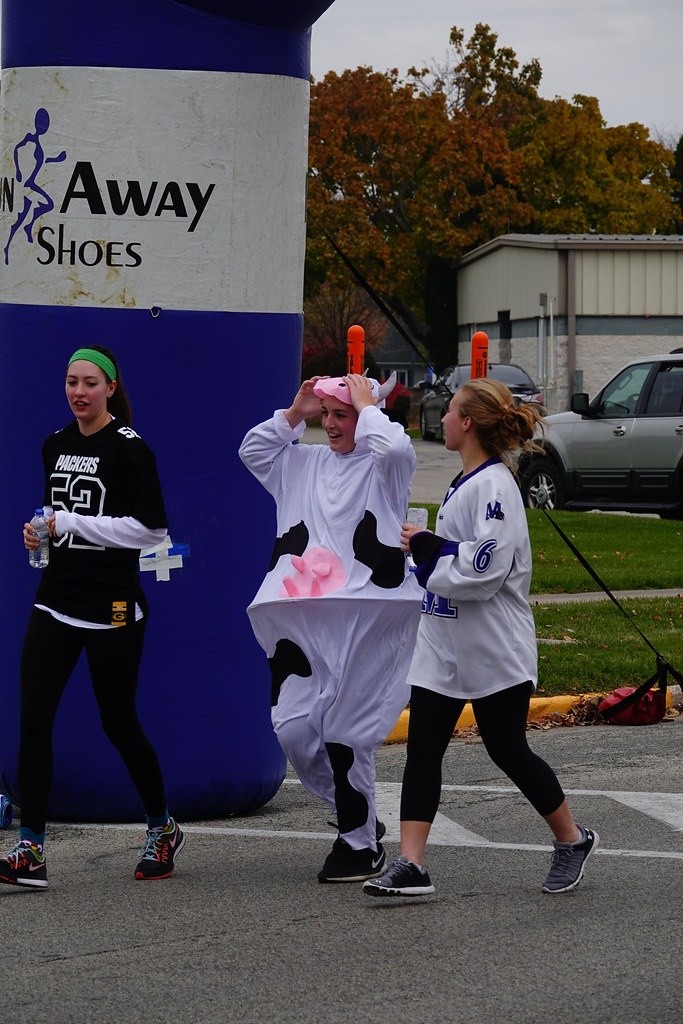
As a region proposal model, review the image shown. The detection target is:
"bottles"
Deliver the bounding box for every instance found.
[28,509,50,568]
[404,507,428,573]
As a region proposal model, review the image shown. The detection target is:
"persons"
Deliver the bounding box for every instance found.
[362,378,599,898]
[237,368,425,885]
[0,343,186,889]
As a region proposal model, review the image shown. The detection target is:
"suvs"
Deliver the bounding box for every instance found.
[515,346,683,521]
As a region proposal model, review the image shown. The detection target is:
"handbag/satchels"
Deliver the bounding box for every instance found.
[598,652,668,726]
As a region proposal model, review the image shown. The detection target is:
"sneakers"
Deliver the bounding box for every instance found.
[134,816,185,880]
[375,819,385,841]
[362,856,435,897]
[542,824,599,892]
[0,839,49,887]
[317,835,388,883]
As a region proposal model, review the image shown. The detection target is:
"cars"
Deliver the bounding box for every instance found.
[418,363,543,444]
